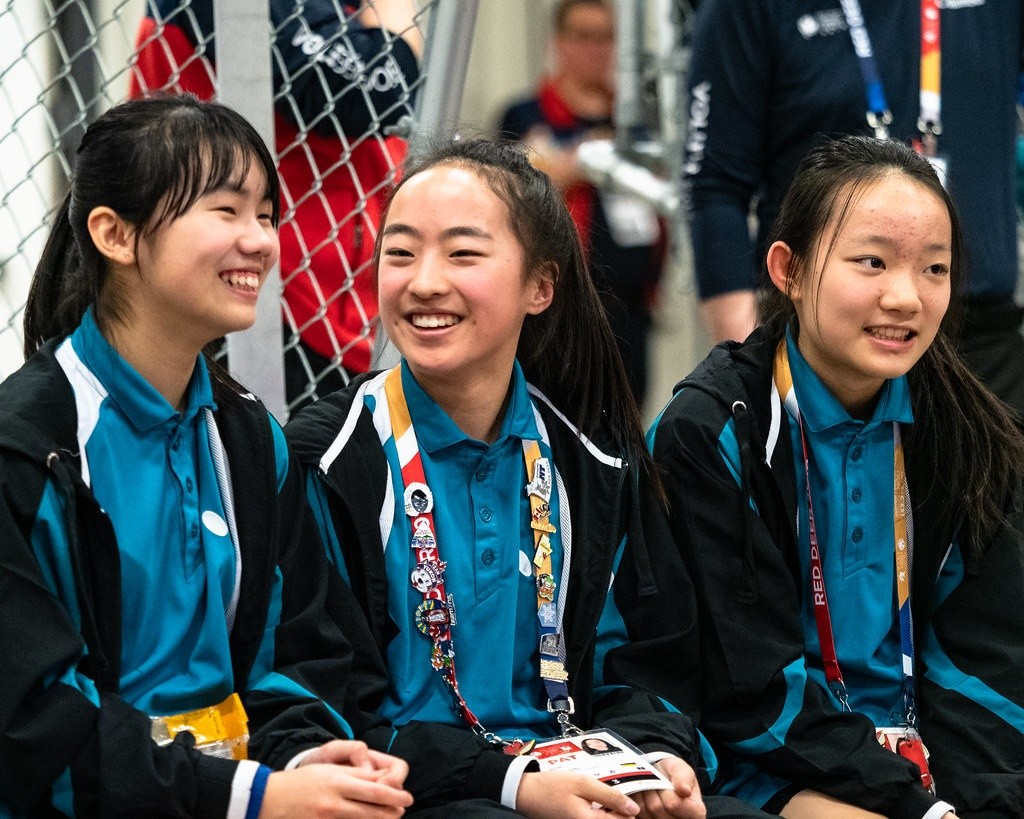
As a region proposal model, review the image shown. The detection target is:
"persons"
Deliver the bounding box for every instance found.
[0,93,412,818]
[581,738,622,755]
[624,137,1024,815]
[274,137,703,818]
[679,2,1021,464]
[487,0,677,459]
[133,0,427,444]
